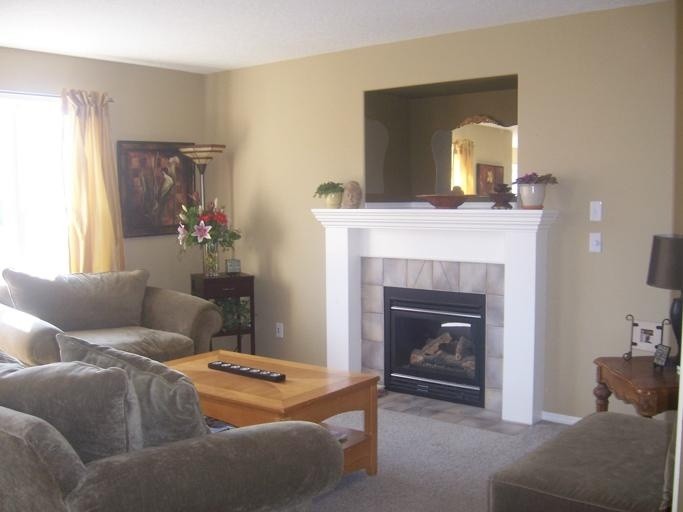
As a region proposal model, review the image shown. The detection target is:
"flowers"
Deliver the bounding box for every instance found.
[178,193,241,271]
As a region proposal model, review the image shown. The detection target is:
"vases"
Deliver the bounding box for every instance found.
[202,242,218,277]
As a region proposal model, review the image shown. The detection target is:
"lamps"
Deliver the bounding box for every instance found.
[647,235,682,365]
[178,143,226,209]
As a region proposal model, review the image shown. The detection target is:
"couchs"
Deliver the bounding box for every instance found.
[0,269,223,367]
[487,333,683,512]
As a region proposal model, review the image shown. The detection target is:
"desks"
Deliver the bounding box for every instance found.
[191,272,255,354]
[593,356,679,418]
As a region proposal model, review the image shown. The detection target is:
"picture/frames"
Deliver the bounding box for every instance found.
[116,141,194,238]
[477,164,503,195]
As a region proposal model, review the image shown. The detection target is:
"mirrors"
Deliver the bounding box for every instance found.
[430,115,518,202]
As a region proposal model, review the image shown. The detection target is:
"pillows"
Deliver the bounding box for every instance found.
[0,269,150,330]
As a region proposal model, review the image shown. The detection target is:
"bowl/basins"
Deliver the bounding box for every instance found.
[416,194,479,209]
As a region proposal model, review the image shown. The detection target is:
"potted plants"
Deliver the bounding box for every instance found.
[220,230,241,274]
[512,172,559,209]
[313,181,344,209]
